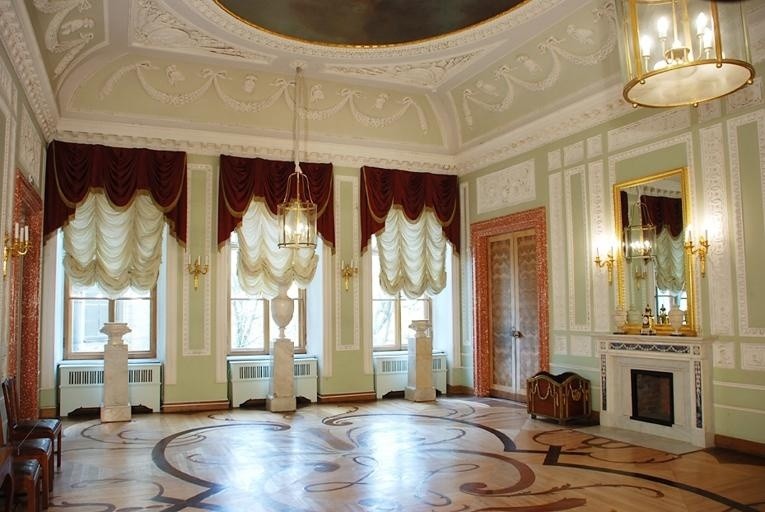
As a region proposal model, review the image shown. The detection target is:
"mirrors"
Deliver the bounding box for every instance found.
[612,167,699,335]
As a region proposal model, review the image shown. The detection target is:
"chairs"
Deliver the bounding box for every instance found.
[0,377,63,512]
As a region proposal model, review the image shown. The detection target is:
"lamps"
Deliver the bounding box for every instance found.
[2,222,30,276]
[686,227,710,278]
[275,66,317,248]
[340,258,358,292]
[615,1,756,110]
[186,254,209,291]
